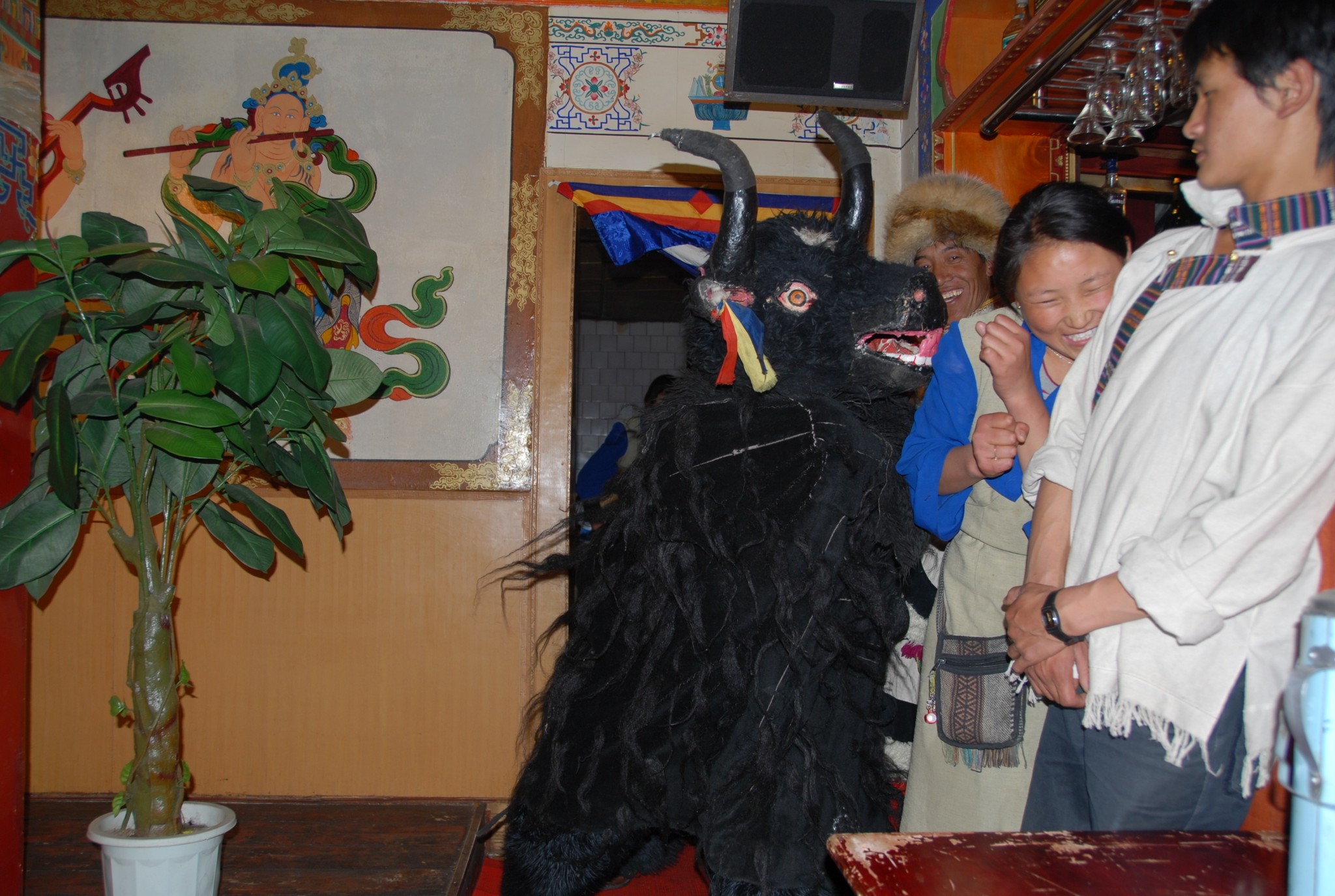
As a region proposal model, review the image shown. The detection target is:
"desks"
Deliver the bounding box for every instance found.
[825,828,1291,896]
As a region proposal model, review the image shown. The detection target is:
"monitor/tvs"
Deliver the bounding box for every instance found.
[723,0,925,112]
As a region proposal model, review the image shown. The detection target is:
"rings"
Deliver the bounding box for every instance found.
[993,444,999,461]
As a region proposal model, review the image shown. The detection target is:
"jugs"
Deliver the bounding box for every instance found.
[1286,589,1335,896]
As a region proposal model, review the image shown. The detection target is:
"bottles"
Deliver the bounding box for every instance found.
[1001,0,1032,51]
[1100,157,1127,217]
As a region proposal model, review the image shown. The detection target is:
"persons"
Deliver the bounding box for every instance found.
[882,0,1335,829]
[574,375,675,599]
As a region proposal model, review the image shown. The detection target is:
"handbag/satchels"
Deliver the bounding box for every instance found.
[924,632,1030,773]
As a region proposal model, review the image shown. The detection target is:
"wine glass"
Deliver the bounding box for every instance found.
[1065,8,1196,148]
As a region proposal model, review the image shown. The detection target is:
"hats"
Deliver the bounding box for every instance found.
[882,171,1010,267]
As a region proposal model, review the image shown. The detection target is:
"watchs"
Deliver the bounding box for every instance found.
[1040,585,1089,647]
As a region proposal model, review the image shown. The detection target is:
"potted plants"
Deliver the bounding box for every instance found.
[0,176,386,896]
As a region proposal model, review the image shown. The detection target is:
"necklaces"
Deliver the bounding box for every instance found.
[1045,345,1076,365]
